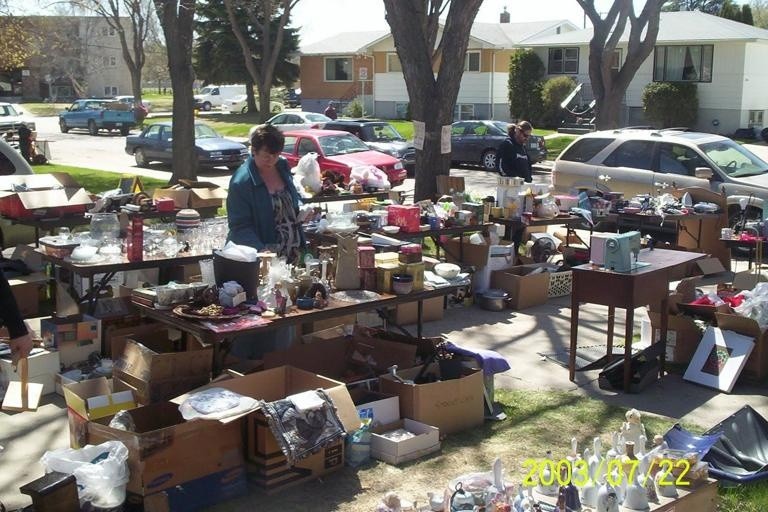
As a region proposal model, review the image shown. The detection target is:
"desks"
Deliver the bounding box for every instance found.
[531,478,718,512]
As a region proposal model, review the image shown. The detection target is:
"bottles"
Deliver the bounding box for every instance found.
[353,184,364,194]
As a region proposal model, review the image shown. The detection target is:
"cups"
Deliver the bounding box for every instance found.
[609,191,624,213]
[59,212,229,264]
[429,217,440,230]
[155,197,175,212]
[721,228,732,239]
[391,273,412,294]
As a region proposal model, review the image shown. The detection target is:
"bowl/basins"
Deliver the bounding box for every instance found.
[433,263,461,279]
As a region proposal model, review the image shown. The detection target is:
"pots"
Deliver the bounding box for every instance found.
[474,289,512,311]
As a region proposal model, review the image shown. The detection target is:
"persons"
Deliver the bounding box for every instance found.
[2,131,14,144]
[325,101,338,120]
[0,268,33,363]
[495,121,545,183]
[221,126,307,362]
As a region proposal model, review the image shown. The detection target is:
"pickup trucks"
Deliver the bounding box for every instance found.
[58,99,137,137]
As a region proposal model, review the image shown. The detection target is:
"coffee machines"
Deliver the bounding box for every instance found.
[496,176,560,221]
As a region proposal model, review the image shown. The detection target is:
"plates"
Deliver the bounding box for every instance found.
[174,302,247,320]
[326,209,401,234]
[62,357,114,382]
[623,194,651,214]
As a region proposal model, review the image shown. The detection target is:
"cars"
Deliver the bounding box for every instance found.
[248,110,332,145]
[222,95,286,115]
[450,119,546,173]
[284,87,301,108]
[125,121,249,173]
[279,129,408,193]
[0,102,38,143]
[112,95,151,111]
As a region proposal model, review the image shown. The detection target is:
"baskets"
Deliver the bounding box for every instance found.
[548,270,573,298]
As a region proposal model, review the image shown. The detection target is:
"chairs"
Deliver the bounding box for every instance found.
[681,148,703,175]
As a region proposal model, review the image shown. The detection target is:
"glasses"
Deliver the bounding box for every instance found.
[523,134,529,138]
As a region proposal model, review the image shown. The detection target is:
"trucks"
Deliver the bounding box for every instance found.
[193,83,247,112]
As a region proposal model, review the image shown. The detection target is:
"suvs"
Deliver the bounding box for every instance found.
[550,126,767,260]
[320,118,416,179]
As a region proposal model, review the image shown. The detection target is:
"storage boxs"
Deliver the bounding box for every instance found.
[647,271,767,395]
